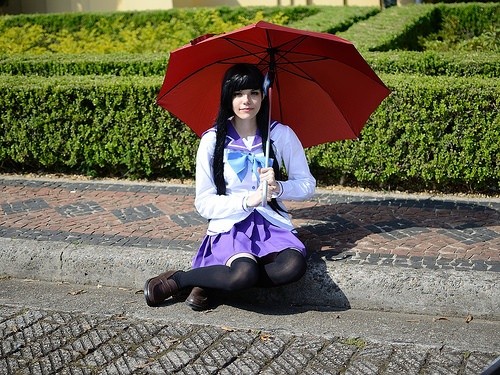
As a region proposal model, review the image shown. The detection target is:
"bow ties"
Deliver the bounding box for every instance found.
[228,151,274,184]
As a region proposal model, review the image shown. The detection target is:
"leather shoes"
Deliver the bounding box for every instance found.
[184,286,210,310]
[143,270,184,306]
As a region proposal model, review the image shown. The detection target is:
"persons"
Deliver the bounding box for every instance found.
[143,61,319,311]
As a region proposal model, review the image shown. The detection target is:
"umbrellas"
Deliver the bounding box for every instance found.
[153,19,395,209]
